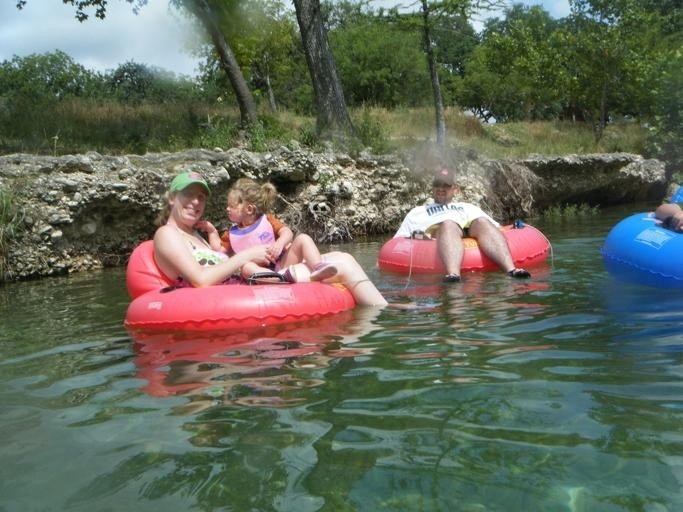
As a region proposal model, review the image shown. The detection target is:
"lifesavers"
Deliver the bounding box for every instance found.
[602,212,682,288]
[378,224,550,273]
[123,240,356,331]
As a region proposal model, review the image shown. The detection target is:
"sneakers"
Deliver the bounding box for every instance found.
[281,264,297,283]
[310,262,338,281]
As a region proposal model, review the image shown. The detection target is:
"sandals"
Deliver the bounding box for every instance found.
[442,272,462,282]
[507,267,529,277]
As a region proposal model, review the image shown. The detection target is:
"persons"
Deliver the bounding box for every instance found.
[152,170,418,309]
[190,176,337,284]
[430,286,514,385]
[374,165,533,282]
[149,332,345,446]
[654,182,683,234]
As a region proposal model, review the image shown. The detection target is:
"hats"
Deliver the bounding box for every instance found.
[169,171,211,199]
[432,168,455,186]
